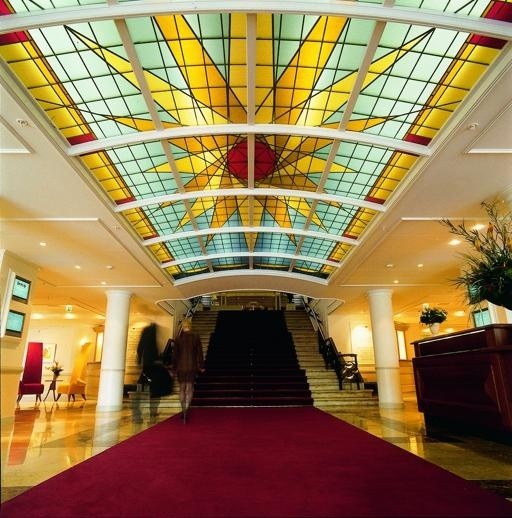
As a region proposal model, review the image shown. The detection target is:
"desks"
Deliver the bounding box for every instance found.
[43,380,63,401]
[43,401,55,418]
[411,324,512,449]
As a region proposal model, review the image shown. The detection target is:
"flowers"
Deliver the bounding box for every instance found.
[437,200,512,324]
[45,361,64,375]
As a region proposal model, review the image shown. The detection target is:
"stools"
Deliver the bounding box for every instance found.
[54,384,88,402]
[16,380,44,404]
[63,402,86,413]
[15,403,40,419]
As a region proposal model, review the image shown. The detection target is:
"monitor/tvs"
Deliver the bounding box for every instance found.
[470,308,491,327]
[12,276,32,304]
[7,308,26,338]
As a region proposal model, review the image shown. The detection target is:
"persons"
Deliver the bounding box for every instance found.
[136,322,158,416]
[173,319,206,424]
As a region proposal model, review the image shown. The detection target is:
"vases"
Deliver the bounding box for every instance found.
[53,373,58,380]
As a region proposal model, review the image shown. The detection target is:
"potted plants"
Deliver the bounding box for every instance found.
[419,305,448,336]
